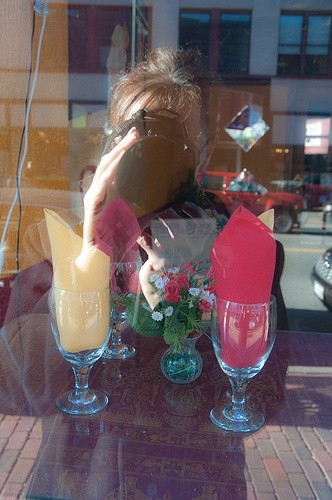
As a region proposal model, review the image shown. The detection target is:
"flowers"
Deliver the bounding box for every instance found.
[148,266,216,338]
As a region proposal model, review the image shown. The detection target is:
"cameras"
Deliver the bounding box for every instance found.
[115,119,199,210]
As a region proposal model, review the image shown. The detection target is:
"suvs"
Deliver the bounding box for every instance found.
[309,246,332,315]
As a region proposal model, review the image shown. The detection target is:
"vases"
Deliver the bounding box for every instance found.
[160,331,204,385]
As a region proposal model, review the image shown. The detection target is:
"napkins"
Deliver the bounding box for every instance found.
[43,208,110,353]
[210,205,276,369]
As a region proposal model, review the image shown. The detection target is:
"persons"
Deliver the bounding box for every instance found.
[0,47,285,500]
[294,173,302,181]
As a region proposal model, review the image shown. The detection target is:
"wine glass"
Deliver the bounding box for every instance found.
[102,255,142,359]
[210,294,277,434]
[49,286,113,415]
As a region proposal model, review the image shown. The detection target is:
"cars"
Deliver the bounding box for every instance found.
[196,170,306,234]
